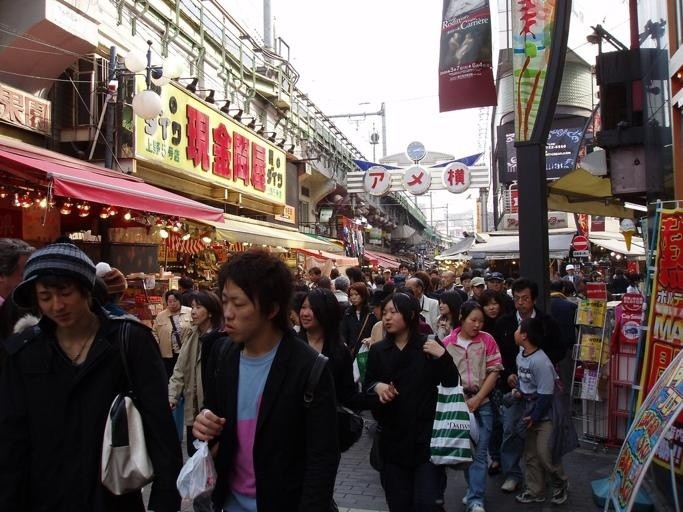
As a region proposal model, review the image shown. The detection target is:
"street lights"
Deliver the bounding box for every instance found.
[95,37,164,270]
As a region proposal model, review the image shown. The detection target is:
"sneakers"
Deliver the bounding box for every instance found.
[461,461,569,512]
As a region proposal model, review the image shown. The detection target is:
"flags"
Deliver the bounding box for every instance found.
[439,1,497,113]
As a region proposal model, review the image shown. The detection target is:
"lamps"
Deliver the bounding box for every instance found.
[109,40,183,121]
[0,184,183,231]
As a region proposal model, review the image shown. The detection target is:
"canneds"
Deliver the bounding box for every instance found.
[159,266,164,278]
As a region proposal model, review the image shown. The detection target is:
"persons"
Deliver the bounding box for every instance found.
[0,233,647,512]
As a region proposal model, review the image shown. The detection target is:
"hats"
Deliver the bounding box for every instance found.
[565,264,574,271]
[11,242,97,313]
[96,260,127,293]
[487,272,503,281]
[383,268,391,274]
[469,277,485,286]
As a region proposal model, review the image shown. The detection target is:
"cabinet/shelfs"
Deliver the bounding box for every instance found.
[568,281,644,452]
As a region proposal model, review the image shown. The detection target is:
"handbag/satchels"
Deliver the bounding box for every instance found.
[369,436,385,470]
[356,343,369,384]
[428,373,474,470]
[337,410,363,451]
[98,320,158,500]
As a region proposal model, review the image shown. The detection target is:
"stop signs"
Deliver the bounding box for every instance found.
[571,235,587,251]
